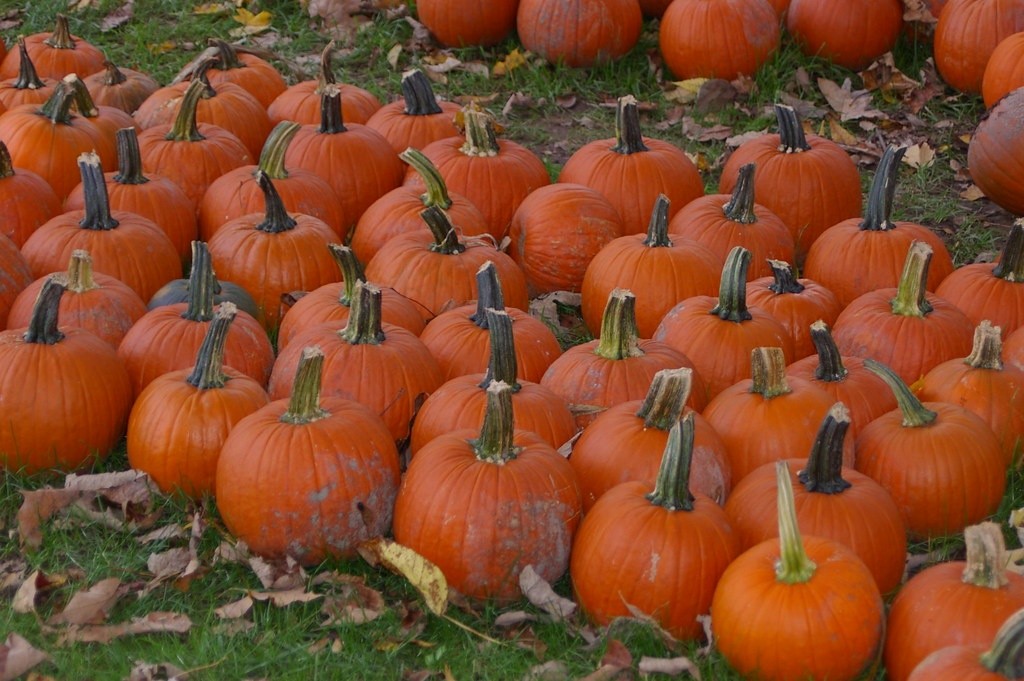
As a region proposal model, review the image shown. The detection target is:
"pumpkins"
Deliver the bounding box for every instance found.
[0,0,1024,681]
